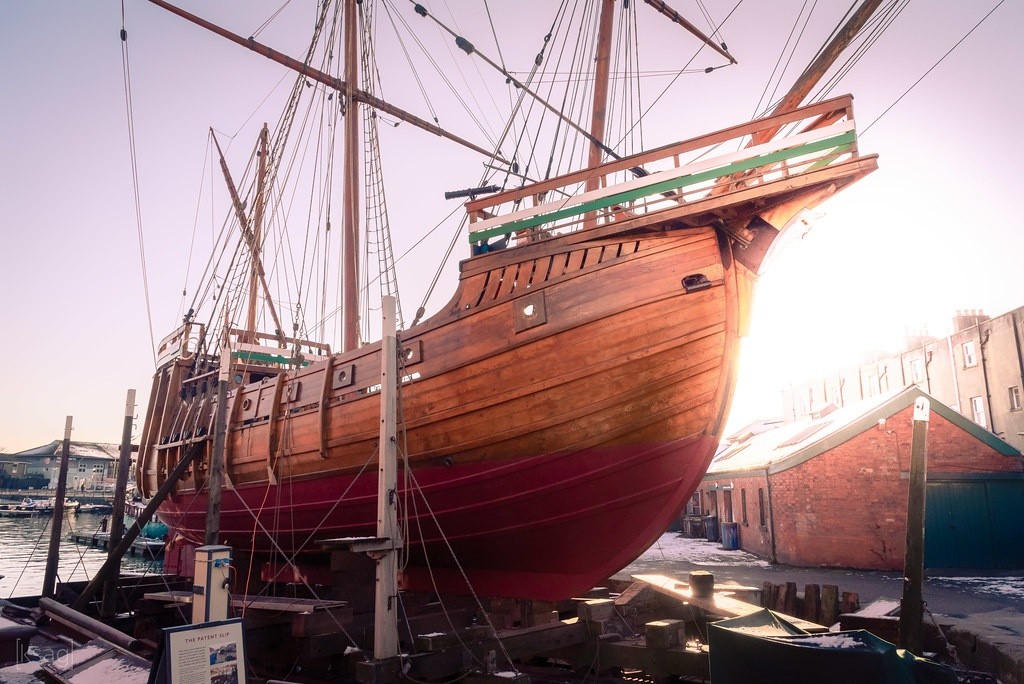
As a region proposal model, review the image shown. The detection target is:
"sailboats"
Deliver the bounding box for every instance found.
[0,1,1021,683]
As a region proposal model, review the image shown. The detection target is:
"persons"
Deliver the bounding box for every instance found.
[209,644,231,665]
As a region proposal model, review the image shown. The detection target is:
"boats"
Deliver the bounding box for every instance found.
[78,502,112,514]
[1,497,79,515]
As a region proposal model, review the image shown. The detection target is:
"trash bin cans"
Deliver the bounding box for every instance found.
[721,523,738,550]
[704,517,719,542]
